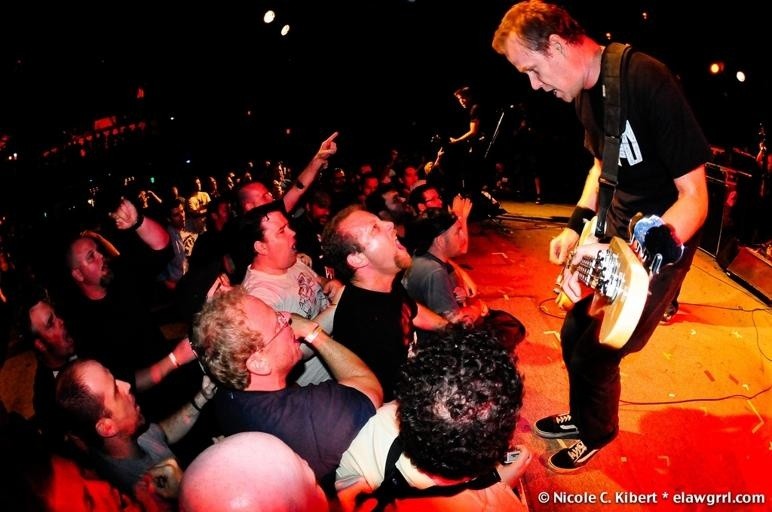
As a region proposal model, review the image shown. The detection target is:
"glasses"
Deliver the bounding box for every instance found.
[263,311,288,347]
[426,196,440,203]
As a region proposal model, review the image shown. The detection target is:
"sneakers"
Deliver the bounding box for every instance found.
[548,441,599,472]
[534,414,583,439]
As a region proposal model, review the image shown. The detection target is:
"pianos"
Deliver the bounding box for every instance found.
[705,144,762,176]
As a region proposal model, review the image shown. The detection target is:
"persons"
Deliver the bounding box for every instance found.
[494,0,711,473]
[0,129,529,512]
[446,87,490,223]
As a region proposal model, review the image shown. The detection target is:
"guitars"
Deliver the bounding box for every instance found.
[553,213,664,350]
[431,133,493,165]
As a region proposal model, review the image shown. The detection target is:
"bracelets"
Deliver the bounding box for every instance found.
[565,203,596,235]
